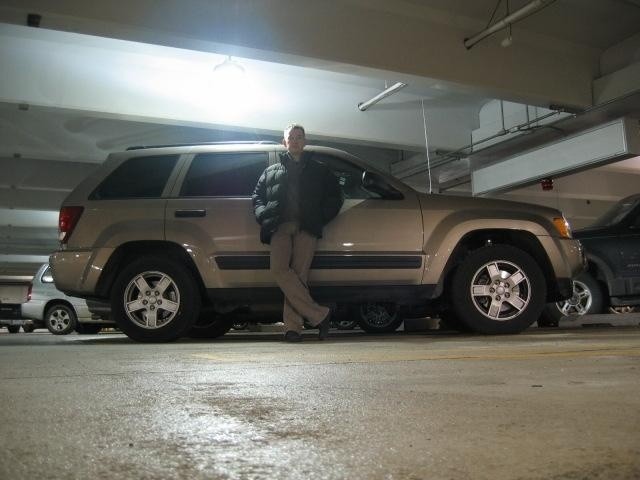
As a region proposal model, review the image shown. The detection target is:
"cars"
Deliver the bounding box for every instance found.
[231,321,360,331]
[609,306,636,314]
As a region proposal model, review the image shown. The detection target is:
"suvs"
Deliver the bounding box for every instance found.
[48,140,589,343]
[547,193,640,317]
[21,264,121,335]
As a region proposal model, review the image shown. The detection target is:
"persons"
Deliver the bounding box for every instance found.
[249,123,345,343]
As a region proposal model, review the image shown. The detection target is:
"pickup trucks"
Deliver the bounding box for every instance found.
[0,299,35,333]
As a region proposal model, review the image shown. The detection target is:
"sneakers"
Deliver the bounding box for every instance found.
[319,306,334,340]
[285,329,302,342]
